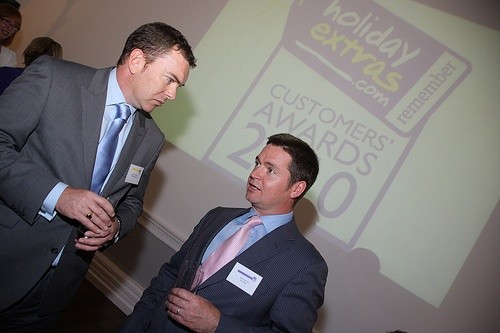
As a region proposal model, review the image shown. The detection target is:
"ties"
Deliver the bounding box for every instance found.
[189,215,263,291]
[90,103,131,194]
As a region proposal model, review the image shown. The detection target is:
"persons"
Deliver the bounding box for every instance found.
[0,21,196,333]
[115,133,328,333]
[0,0,23,68]
[0,37,63,95]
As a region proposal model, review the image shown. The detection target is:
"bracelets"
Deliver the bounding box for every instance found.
[114,218,120,239]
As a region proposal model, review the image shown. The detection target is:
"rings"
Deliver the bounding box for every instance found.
[177,308,181,314]
[87,212,94,220]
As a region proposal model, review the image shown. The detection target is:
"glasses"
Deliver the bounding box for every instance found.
[0,16,20,31]
